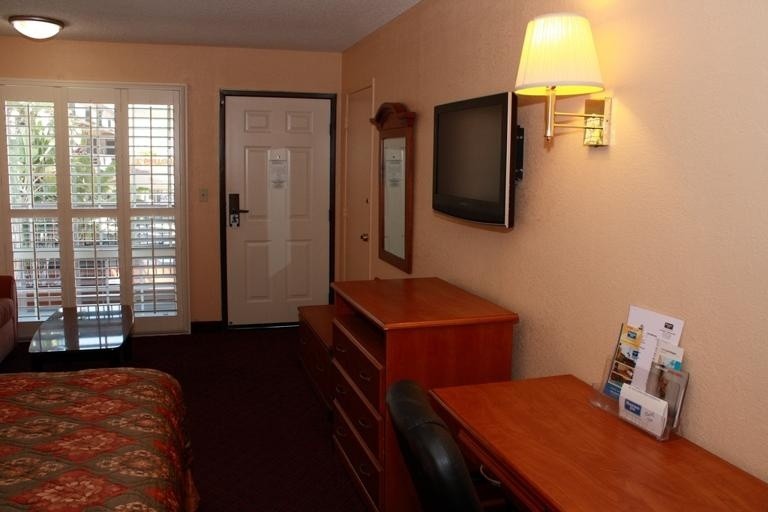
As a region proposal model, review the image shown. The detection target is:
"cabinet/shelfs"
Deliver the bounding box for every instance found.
[297,304,338,457]
[327,278,517,510]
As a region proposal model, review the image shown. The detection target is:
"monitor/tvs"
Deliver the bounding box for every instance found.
[432,90,524,229]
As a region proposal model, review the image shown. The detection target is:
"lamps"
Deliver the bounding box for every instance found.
[514,13,613,148]
[8,14,64,41]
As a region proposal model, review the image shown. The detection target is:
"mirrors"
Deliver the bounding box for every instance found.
[368,102,416,274]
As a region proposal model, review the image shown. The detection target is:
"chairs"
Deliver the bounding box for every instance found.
[1,275,19,362]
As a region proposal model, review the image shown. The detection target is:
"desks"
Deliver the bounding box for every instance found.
[27,303,135,371]
[426,373,767,512]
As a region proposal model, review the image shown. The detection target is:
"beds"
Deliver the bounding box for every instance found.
[1,368,188,512]
